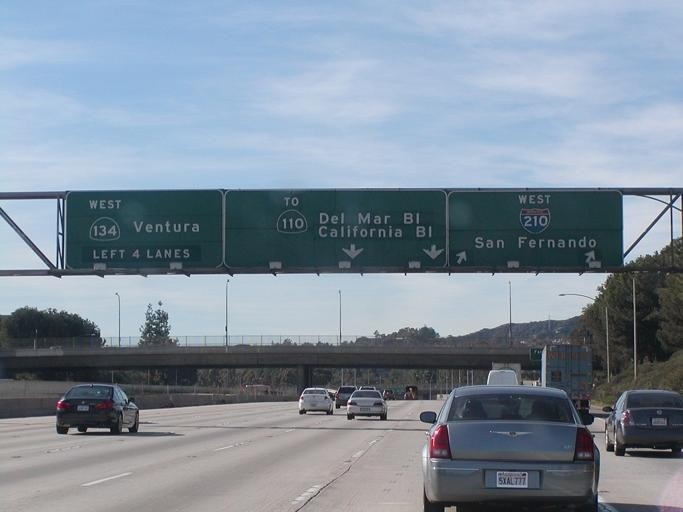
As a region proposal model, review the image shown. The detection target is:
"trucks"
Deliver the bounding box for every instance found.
[540,342,594,417]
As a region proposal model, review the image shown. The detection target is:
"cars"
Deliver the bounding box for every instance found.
[418,383,601,512]
[55,382,140,435]
[298,385,395,421]
[601,389,683,457]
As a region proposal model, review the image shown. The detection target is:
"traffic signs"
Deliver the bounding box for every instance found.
[448,190,624,269]
[223,189,446,270]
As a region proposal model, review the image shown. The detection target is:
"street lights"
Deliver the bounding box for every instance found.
[558,292,611,386]
[115,291,121,347]
[338,289,342,348]
[508,279,512,344]
[225,279,230,350]
